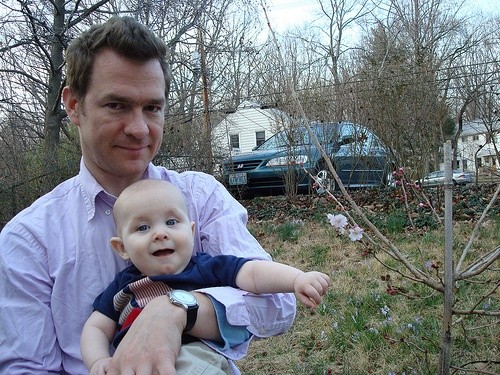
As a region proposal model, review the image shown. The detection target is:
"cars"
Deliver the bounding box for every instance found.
[220,121,399,201]
[414,171,476,187]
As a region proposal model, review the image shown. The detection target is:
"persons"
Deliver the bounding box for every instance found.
[0,16,297,375]
[80,179,330,375]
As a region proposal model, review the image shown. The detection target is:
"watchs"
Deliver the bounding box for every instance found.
[166,290,199,333]
